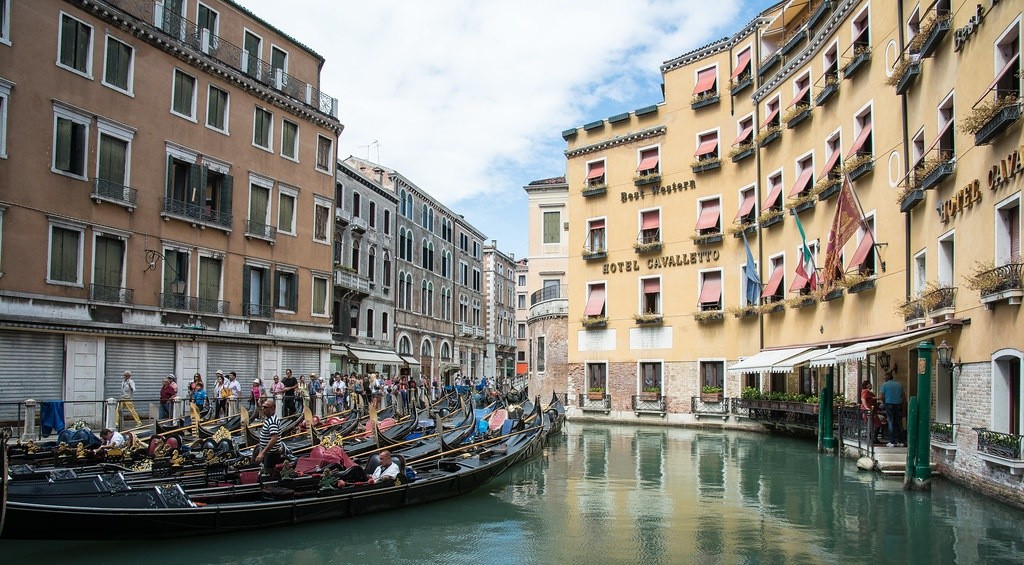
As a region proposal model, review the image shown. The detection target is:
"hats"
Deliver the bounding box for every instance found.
[253,378,260,384]
[214,370,223,375]
[166,373,175,380]
[309,373,316,377]
[123,370,132,376]
[401,375,406,378]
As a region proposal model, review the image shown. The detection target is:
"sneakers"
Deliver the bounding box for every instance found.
[896,443,904,447]
[886,442,895,447]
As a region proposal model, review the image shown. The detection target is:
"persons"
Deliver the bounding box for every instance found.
[327,372,428,414]
[159,374,178,421]
[249,379,267,406]
[880,374,905,446]
[270,369,298,416]
[496,376,510,389]
[213,370,242,419]
[433,378,438,398]
[190,373,206,412]
[93,429,125,452]
[455,374,496,392]
[115,371,142,427]
[367,450,400,481]
[256,401,282,479]
[861,381,882,444]
[296,374,328,413]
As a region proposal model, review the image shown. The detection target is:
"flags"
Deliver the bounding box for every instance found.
[823,178,860,295]
[744,241,761,305]
[794,214,817,290]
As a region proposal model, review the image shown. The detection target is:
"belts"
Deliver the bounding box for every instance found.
[310,393,316,395]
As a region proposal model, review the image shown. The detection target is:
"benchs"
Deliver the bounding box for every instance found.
[360,454,407,485]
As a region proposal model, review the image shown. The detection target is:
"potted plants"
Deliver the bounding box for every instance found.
[578,7,1024,451]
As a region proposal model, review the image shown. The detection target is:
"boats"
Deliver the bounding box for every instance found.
[537,390,567,438]
[0,396,544,528]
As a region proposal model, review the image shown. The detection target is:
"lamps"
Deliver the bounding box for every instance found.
[878,351,897,377]
[934,339,963,374]
[142,247,187,293]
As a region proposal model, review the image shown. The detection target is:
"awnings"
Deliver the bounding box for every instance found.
[330,345,348,356]
[727,346,842,374]
[584,51,1020,315]
[402,356,421,368]
[350,347,404,366]
[809,323,953,367]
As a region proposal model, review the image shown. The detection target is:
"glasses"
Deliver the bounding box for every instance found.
[310,376,314,377]
[195,376,199,378]
[167,377,173,380]
[261,406,272,409]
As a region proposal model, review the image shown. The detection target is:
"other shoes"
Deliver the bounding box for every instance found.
[874,439,881,444]
[211,421,220,425]
[255,419,260,421]
[135,423,143,427]
